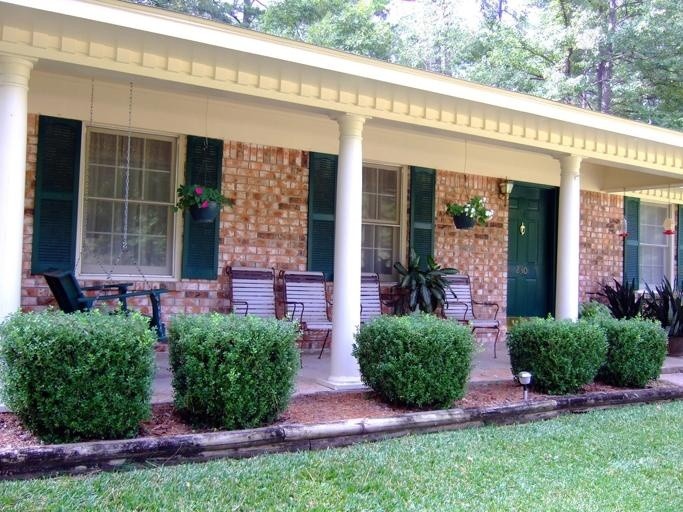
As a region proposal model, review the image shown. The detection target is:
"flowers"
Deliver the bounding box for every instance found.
[444,195,495,226]
[168,184,236,215]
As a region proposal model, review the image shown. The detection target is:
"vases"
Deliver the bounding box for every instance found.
[187,201,220,225]
[451,213,476,229]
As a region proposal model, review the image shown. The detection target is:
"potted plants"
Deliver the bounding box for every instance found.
[645,275,683,356]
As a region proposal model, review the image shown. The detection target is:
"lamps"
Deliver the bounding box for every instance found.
[607,218,630,239]
[497,174,514,208]
[661,182,677,235]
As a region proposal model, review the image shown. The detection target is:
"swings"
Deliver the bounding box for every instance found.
[44,77,169,342]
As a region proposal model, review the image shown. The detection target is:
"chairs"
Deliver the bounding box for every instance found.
[43,270,173,341]
[359,271,382,325]
[441,272,501,359]
[221,266,333,370]
